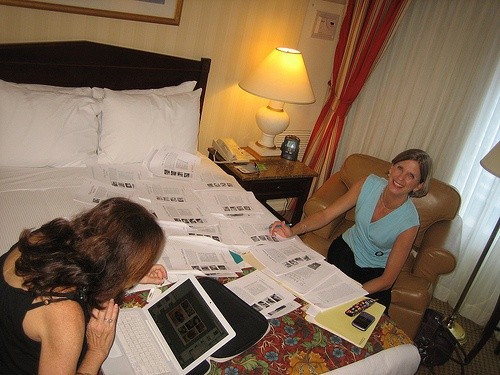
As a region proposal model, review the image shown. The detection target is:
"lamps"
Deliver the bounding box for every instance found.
[239,48,316,157]
[441,142,500,342]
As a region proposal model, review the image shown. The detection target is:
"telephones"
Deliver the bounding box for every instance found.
[212,137,250,164]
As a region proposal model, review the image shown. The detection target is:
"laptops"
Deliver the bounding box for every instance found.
[98,272,236,375]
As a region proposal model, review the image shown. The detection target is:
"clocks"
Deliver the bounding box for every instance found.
[281,134,300,162]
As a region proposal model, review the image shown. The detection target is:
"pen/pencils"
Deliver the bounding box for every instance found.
[265,224,292,229]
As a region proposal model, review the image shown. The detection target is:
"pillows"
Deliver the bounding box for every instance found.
[0,79,99,166]
[94,81,204,161]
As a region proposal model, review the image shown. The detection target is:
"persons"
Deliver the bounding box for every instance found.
[268,148,433,316]
[0,197,168,375]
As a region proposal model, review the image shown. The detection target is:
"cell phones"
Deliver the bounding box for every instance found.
[352,311,375,332]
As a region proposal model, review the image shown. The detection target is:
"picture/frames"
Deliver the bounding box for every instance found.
[0,0,183,26]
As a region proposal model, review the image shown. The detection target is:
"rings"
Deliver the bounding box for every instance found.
[105,318,112,322]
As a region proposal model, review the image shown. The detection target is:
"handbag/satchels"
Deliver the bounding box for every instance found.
[413,319,467,375]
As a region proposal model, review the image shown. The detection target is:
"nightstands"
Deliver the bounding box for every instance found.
[207,147,318,226]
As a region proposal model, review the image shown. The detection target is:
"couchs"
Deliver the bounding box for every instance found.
[301,153,462,340]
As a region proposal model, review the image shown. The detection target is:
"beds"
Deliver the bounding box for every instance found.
[0,40,420,375]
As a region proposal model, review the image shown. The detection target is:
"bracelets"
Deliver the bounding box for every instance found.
[300,222,308,234]
